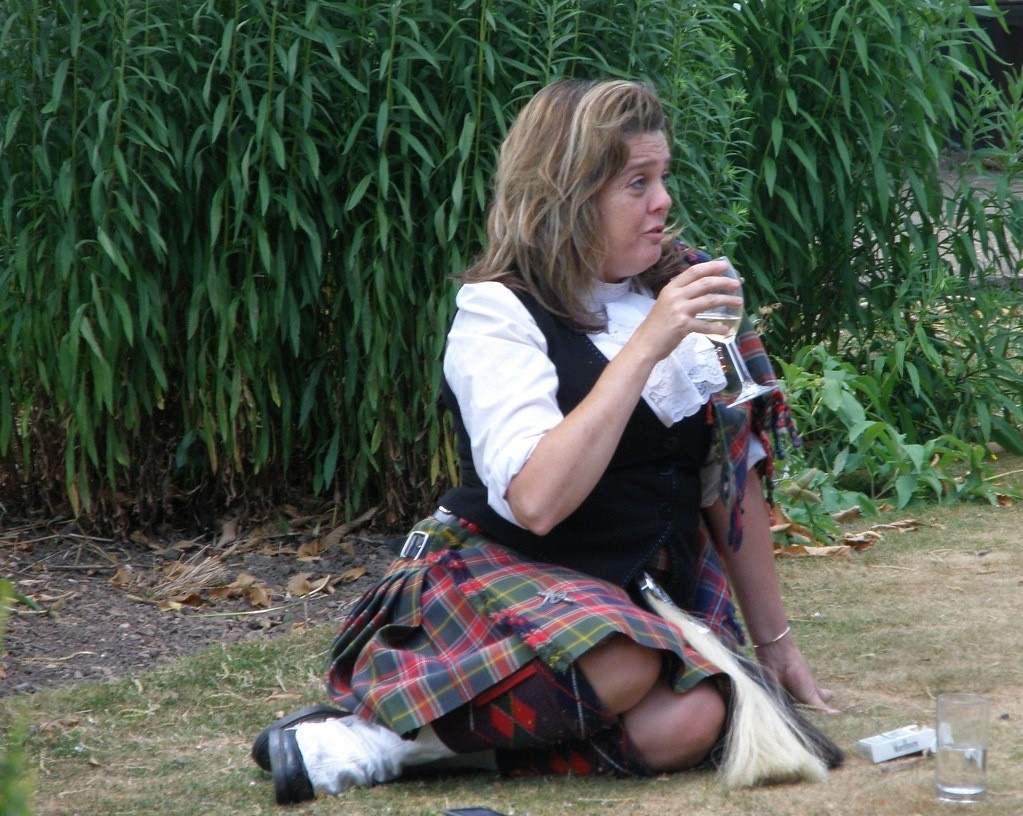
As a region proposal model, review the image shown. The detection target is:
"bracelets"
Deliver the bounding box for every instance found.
[754,627,792,648]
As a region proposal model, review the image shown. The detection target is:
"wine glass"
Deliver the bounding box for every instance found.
[670,255,778,409]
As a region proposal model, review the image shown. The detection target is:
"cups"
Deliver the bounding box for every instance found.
[933,692,993,803]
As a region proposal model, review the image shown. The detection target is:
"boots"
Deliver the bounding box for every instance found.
[251,706,461,804]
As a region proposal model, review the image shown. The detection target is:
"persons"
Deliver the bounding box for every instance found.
[250,77,837,805]
